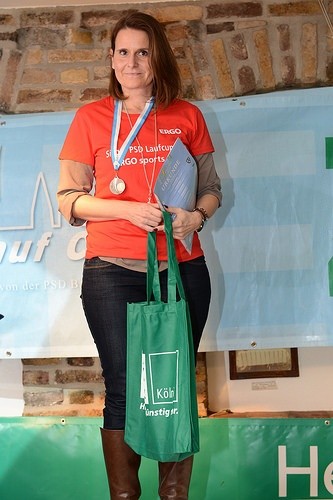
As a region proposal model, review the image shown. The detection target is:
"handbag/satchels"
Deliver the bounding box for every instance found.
[123,209,201,463]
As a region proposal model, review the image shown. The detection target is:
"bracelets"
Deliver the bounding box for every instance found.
[191,205,206,234]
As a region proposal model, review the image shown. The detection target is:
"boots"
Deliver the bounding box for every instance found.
[157,453,194,500]
[99,426,142,500]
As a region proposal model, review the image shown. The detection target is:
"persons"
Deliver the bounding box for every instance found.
[58,10,224,500]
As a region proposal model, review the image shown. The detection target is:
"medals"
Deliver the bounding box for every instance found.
[109,176,126,195]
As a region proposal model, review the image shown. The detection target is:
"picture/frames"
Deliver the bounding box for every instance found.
[229,348,300,381]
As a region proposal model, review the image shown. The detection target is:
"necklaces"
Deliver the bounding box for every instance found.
[121,98,158,204]
